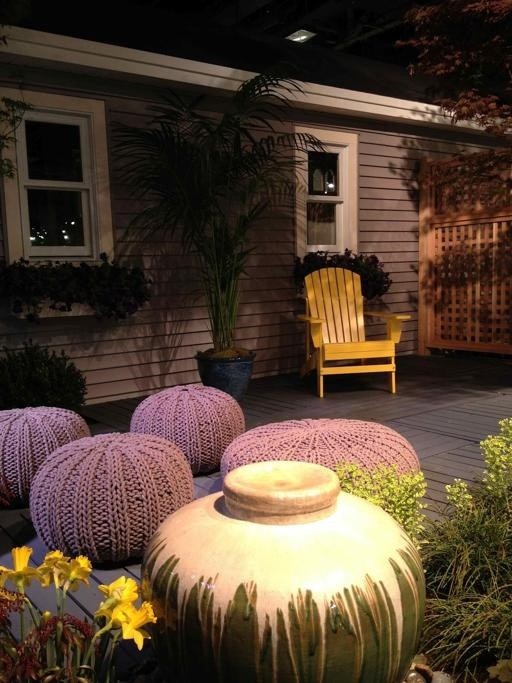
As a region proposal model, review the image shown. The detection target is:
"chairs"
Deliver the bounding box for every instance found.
[296,268,411,397]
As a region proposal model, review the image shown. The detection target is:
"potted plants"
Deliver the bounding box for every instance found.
[109,66,329,402]
